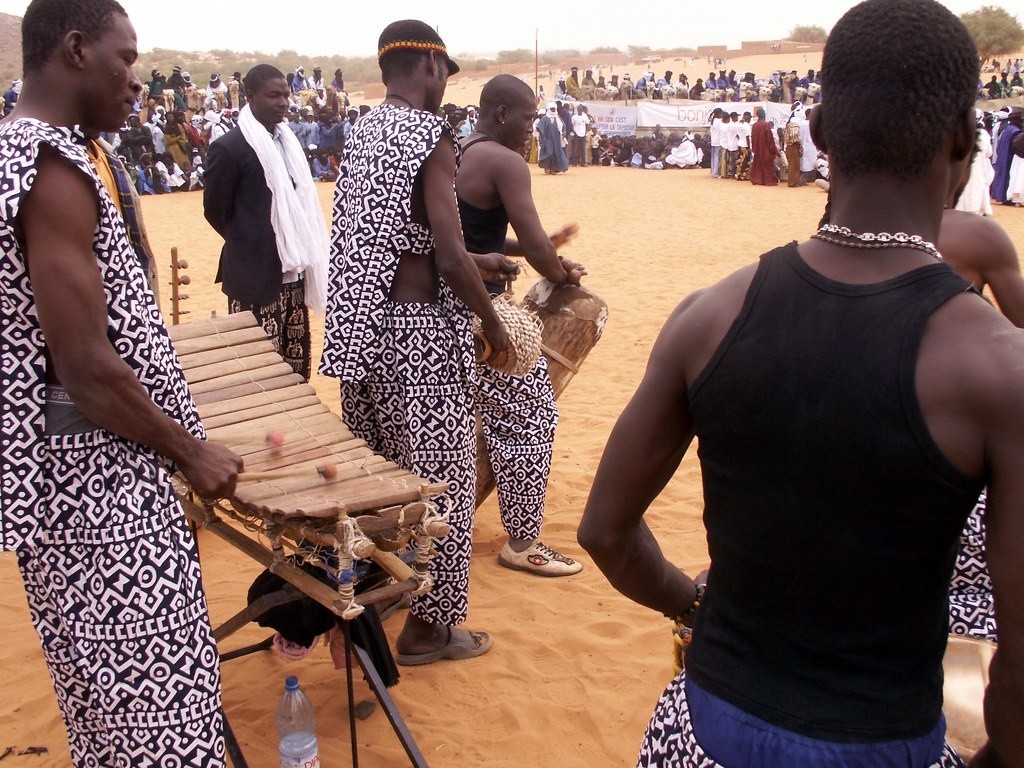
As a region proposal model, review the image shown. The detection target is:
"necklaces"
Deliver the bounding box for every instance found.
[808,225,944,262]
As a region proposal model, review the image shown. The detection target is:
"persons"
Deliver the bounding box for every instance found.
[453,73,589,579]
[74,129,161,316]
[316,19,523,667]
[934,114,1024,645]
[0,0,248,768]
[575,1,1024,768]
[0,38,1024,222]
[201,64,313,387]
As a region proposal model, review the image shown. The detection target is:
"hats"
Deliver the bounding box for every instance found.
[284,65,371,160]
[99,66,249,174]
[712,100,804,120]
[437,103,480,122]
[558,66,822,84]
[11,79,23,94]
[975,106,1024,126]
[536,100,588,116]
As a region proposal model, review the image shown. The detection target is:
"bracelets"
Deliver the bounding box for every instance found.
[661,583,706,624]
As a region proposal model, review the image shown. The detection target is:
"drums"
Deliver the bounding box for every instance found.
[476,272,607,514]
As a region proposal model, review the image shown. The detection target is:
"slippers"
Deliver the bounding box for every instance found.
[378,592,411,622]
[396,627,492,666]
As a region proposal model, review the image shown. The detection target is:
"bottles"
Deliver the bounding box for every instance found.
[278,674,324,768]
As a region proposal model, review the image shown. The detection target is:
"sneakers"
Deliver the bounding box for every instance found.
[498,538,583,577]
